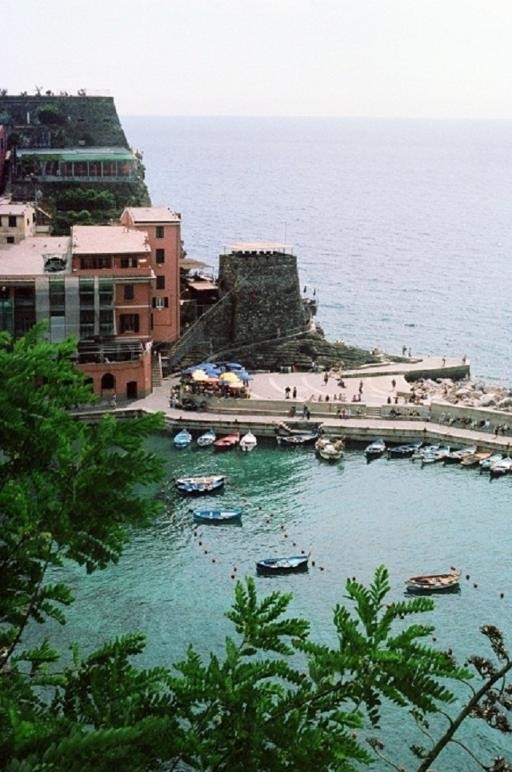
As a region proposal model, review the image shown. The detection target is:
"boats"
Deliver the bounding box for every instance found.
[314,437,343,463]
[363,437,386,456]
[195,429,217,450]
[213,430,241,451]
[401,569,464,595]
[190,508,244,524]
[239,429,258,455]
[274,433,318,445]
[175,474,228,494]
[172,428,193,451]
[386,441,512,478]
[254,555,310,575]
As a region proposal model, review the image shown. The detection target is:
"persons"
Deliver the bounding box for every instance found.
[285,345,467,421]
[439,411,512,438]
[172,382,250,413]
